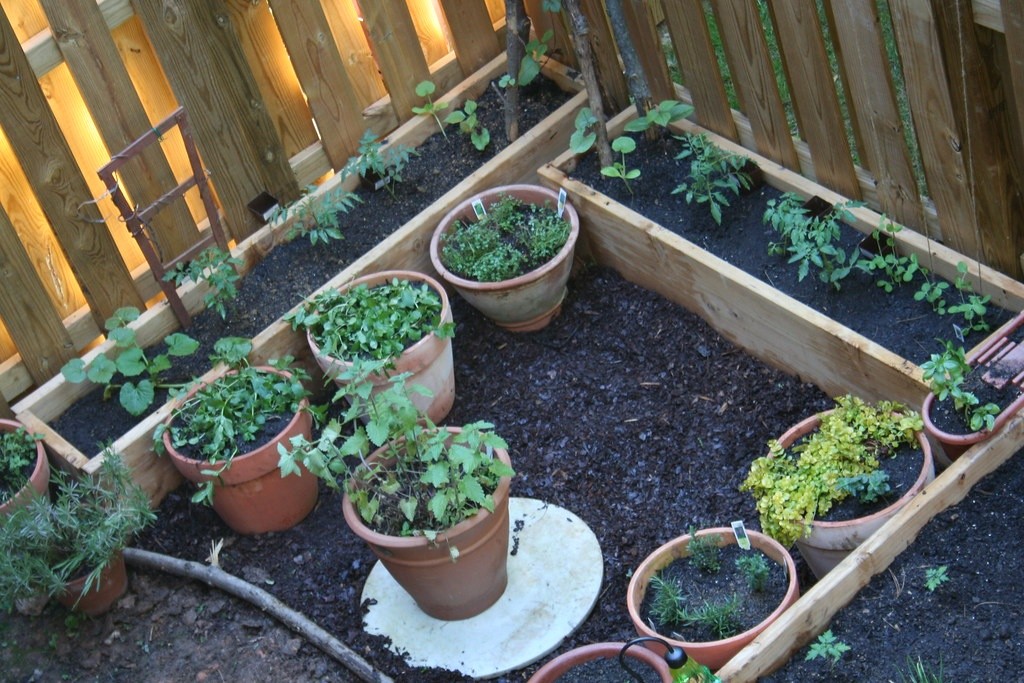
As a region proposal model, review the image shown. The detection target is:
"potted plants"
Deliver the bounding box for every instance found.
[279,270,457,438]
[626,527,800,675]
[429,182,580,334]
[0,419,54,530]
[0,437,162,619]
[698,408,1022,682]
[738,394,935,583]
[535,99,1024,420]
[920,337,1024,463]
[277,352,518,622]
[62,305,318,536]
[6,44,596,533]
[526,637,724,683]
